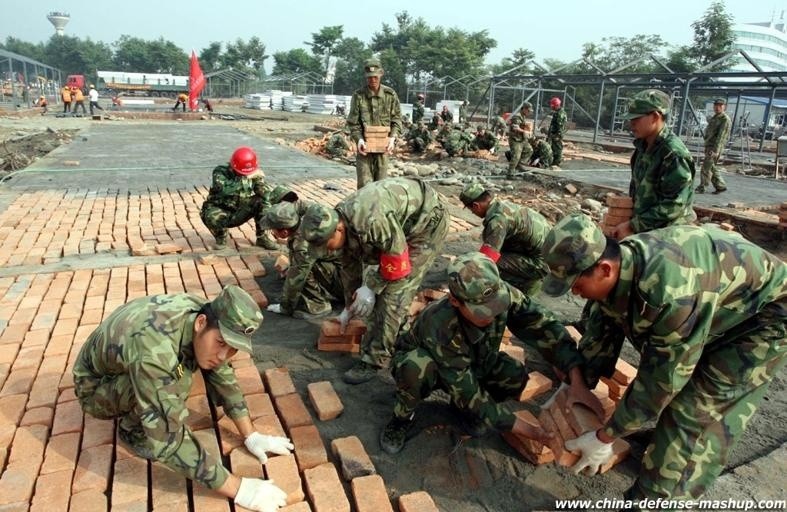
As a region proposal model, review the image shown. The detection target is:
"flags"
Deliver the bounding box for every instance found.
[189,46,209,114]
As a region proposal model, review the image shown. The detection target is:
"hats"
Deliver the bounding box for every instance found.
[418,94,425,98]
[364,60,384,77]
[301,204,340,258]
[447,251,510,321]
[618,89,671,120]
[714,98,727,105]
[270,186,291,204]
[540,213,607,297]
[523,102,531,108]
[211,285,263,354]
[259,204,298,231]
[459,184,485,210]
[477,125,486,130]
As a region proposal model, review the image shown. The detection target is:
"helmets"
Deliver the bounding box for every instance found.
[550,98,561,107]
[231,148,257,174]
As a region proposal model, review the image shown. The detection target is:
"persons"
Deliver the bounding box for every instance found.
[0,76,215,120]
[537,204,787,511]
[71,281,298,511]
[257,60,732,455]
[199,145,279,252]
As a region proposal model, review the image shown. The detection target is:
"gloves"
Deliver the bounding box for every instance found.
[267,304,282,312]
[331,309,351,333]
[564,428,612,479]
[352,286,376,318]
[538,382,571,410]
[245,433,294,465]
[234,477,286,511]
[357,139,367,156]
[386,138,395,153]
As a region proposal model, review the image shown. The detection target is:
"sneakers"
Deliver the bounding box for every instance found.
[345,363,377,383]
[507,175,519,180]
[712,187,726,195]
[291,307,332,321]
[447,396,487,437]
[694,185,705,193]
[380,415,410,453]
[118,423,156,461]
